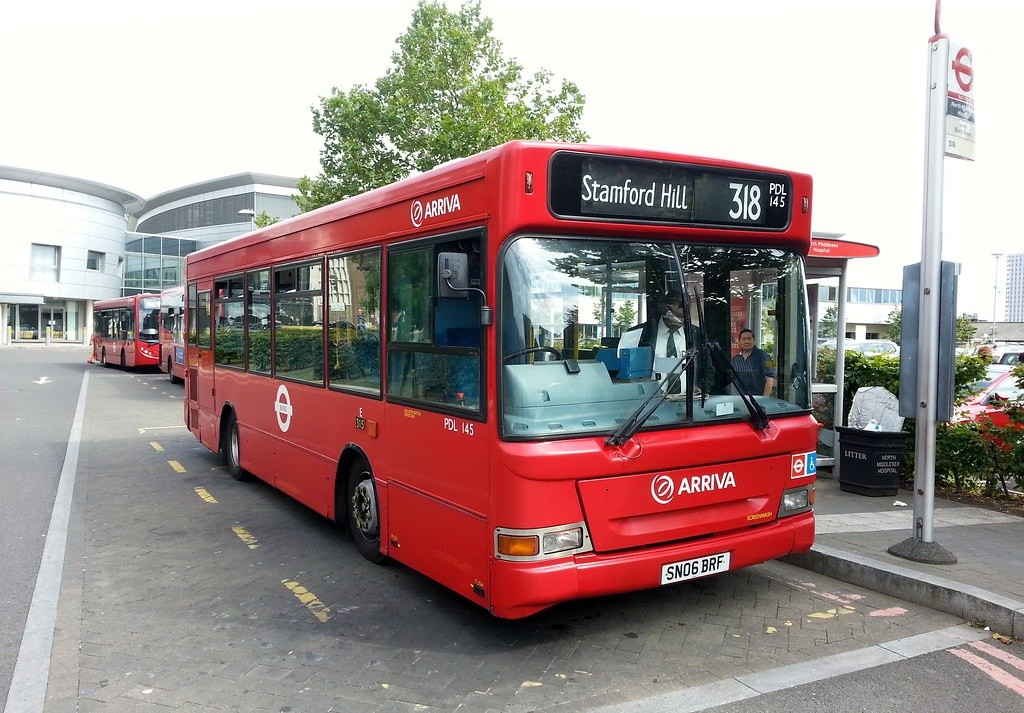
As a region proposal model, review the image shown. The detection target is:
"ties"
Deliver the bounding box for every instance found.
[667,333,681,394]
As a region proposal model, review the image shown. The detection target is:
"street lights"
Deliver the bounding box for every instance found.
[990,252,1004,342]
[237,208,255,231]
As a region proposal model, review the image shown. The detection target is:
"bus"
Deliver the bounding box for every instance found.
[92,292,162,372]
[157,285,185,384]
[183,140,818,621]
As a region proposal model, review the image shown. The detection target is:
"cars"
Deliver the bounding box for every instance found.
[816,335,1024,455]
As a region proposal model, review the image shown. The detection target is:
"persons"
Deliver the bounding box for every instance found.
[620,289,717,401]
[729,324,775,402]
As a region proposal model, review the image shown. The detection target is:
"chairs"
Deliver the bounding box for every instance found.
[213,316,379,390]
